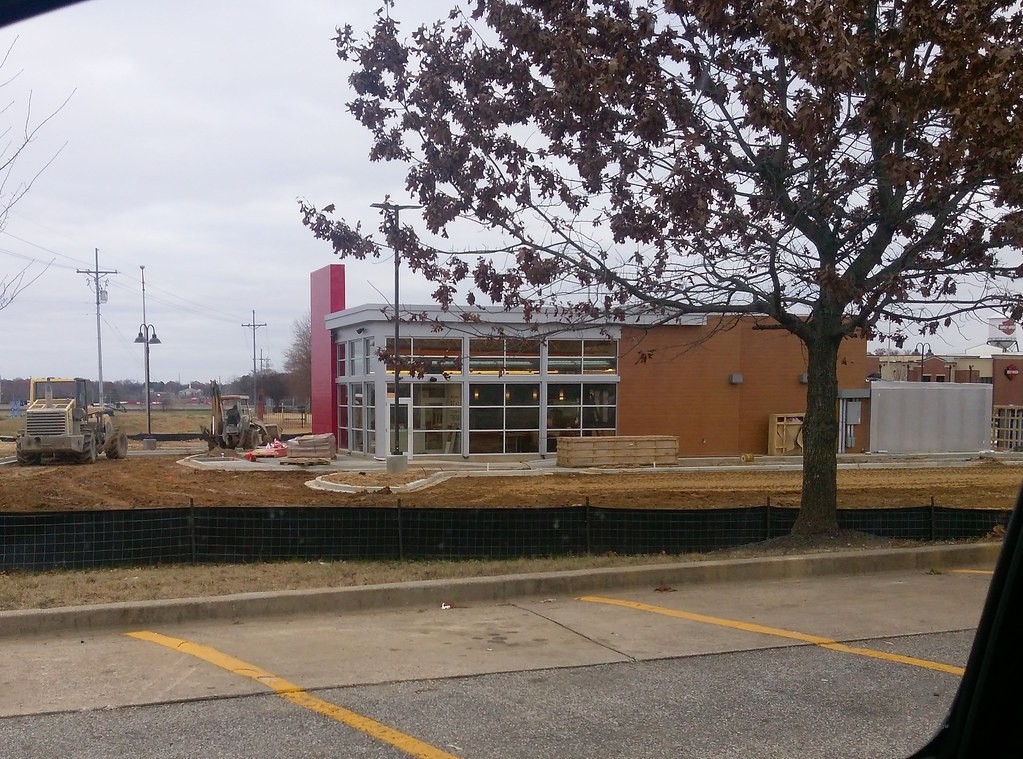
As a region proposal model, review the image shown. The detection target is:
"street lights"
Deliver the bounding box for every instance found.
[135,322,161,439]
[368,200,422,456]
[914,342,932,382]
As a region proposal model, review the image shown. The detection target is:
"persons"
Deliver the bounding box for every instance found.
[225,405,241,426]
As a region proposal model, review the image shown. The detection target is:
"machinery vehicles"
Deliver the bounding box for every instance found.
[14,375,129,466]
[199,378,269,456]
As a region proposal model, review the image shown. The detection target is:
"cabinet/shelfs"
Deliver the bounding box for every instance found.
[769,412,806,456]
[991,403,1022,451]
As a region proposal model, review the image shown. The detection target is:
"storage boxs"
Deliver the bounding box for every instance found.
[556,434,680,471]
